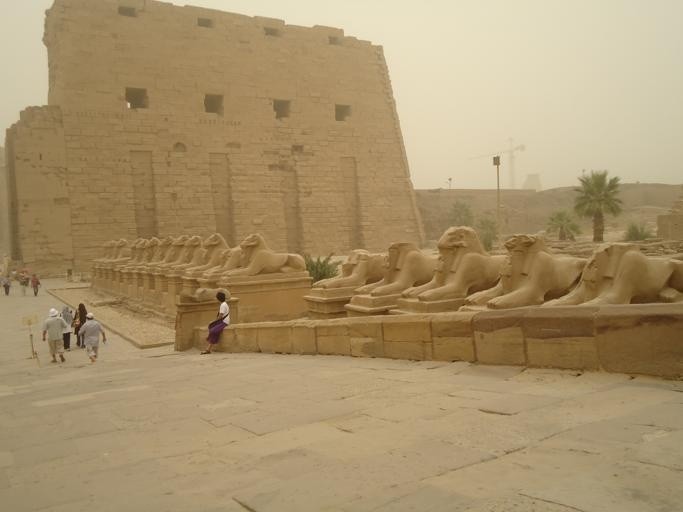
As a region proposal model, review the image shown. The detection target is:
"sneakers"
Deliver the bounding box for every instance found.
[200,350,210,354]
[50,343,86,363]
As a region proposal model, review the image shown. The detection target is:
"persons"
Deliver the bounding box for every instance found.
[0,268,40,296]
[43,303,107,362]
[201,293,230,354]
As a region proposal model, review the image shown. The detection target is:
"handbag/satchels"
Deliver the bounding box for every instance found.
[208,318,222,328]
[71,319,80,327]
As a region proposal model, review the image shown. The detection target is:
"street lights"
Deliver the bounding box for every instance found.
[493,156,500,218]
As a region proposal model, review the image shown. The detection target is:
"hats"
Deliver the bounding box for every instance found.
[85,313,96,318]
[48,308,58,317]
